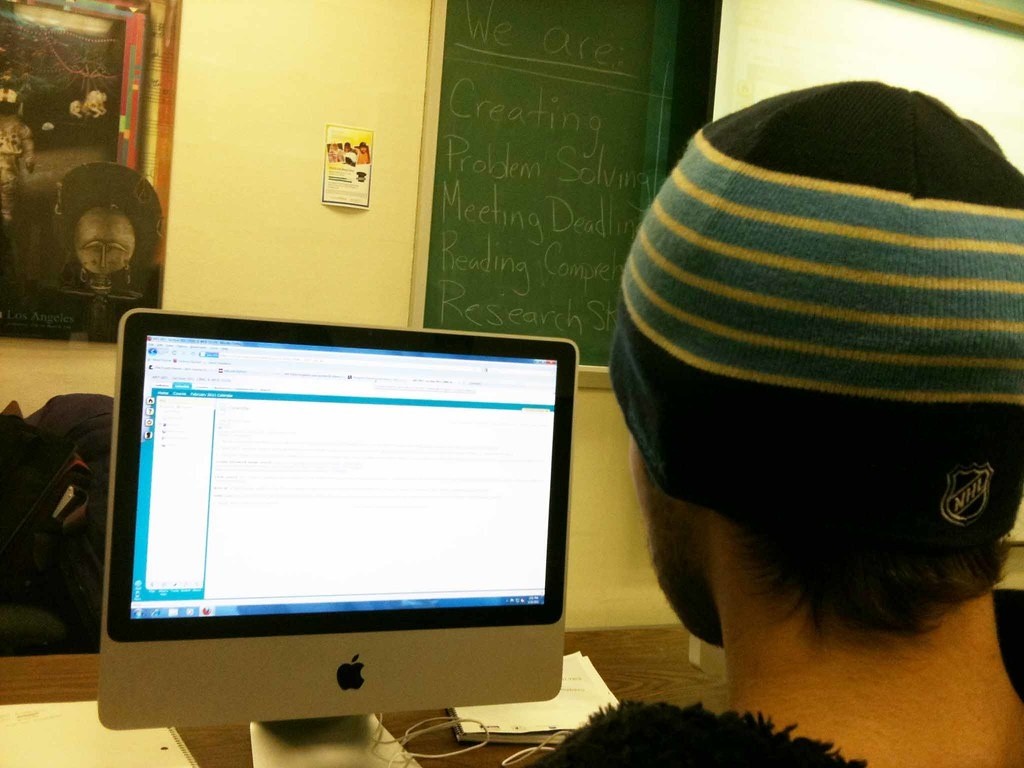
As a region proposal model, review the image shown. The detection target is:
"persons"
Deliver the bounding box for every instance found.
[532,82,1024,768]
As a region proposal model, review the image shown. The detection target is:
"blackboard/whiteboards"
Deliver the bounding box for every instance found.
[406,1,724,391]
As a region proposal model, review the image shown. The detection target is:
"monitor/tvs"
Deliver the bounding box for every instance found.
[98,308,579,768]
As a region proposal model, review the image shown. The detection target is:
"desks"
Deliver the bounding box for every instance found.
[0,624,726,768]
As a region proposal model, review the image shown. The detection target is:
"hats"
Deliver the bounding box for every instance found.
[611,81,1024,548]
